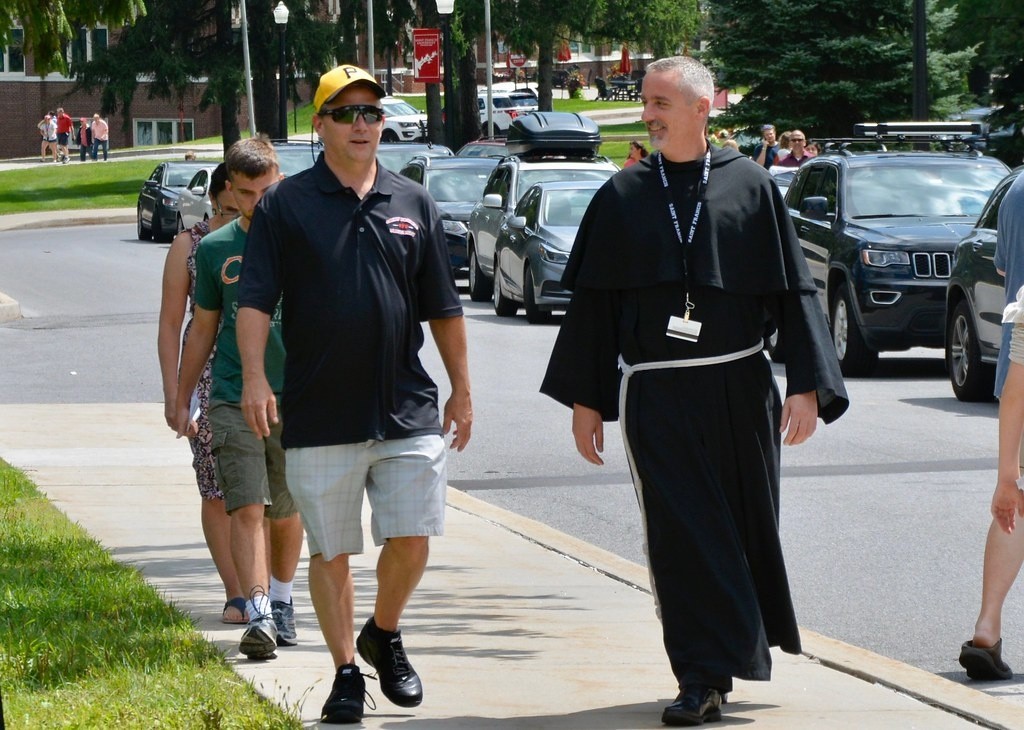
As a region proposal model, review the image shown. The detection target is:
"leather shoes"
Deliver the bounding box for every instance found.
[662,683,721,725]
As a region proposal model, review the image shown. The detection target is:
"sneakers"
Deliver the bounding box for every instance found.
[270,595,298,646]
[239,585,278,659]
[357,618,423,707]
[321,664,377,720]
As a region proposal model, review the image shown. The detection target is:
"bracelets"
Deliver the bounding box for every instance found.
[762,147,766,151]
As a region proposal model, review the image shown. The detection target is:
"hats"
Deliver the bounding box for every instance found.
[79,118,86,122]
[313,65,386,114]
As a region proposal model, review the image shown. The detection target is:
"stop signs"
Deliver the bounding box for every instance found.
[509,47,526,67]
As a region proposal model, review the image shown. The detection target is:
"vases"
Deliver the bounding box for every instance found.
[569,89,581,99]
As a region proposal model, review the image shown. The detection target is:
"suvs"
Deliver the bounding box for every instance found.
[765,121,1013,380]
[466,112,623,303]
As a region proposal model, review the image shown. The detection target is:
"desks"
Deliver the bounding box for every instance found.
[610,81,636,100]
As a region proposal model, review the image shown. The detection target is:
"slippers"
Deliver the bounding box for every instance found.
[222,597,249,623]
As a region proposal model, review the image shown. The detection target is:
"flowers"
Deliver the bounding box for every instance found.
[567,73,585,90]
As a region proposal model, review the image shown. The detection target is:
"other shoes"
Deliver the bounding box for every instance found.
[961,639,1013,679]
[63,157,68,164]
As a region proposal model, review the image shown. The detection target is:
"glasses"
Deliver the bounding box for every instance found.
[213,196,238,222]
[320,104,383,124]
[790,139,803,142]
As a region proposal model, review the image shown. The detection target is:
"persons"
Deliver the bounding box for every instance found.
[773,130,821,168]
[76,117,93,162]
[990,286,1024,534]
[92,113,109,161]
[236,65,474,721]
[958,170,1024,682]
[594,74,603,88]
[722,140,738,150]
[752,125,780,171]
[158,161,271,622]
[185,151,196,161]
[174,138,304,658]
[624,141,648,168]
[539,55,850,725]
[38,111,63,163]
[56,107,76,164]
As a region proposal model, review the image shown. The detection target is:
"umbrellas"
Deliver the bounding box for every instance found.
[557,41,571,69]
[620,42,631,74]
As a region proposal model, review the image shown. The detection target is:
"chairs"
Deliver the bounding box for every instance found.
[581,68,592,89]
[595,70,646,102]
[549,196,570,226]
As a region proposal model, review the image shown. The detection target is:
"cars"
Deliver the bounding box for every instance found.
[945,165,1023,404]
[176,166,215,234]
[271,90,565,278]
[493,180,608,325]
[137,161,223,243]
[937,104,1018,158]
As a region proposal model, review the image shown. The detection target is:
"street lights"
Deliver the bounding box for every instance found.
[273,1,289,142]
[437,0,456,152]
